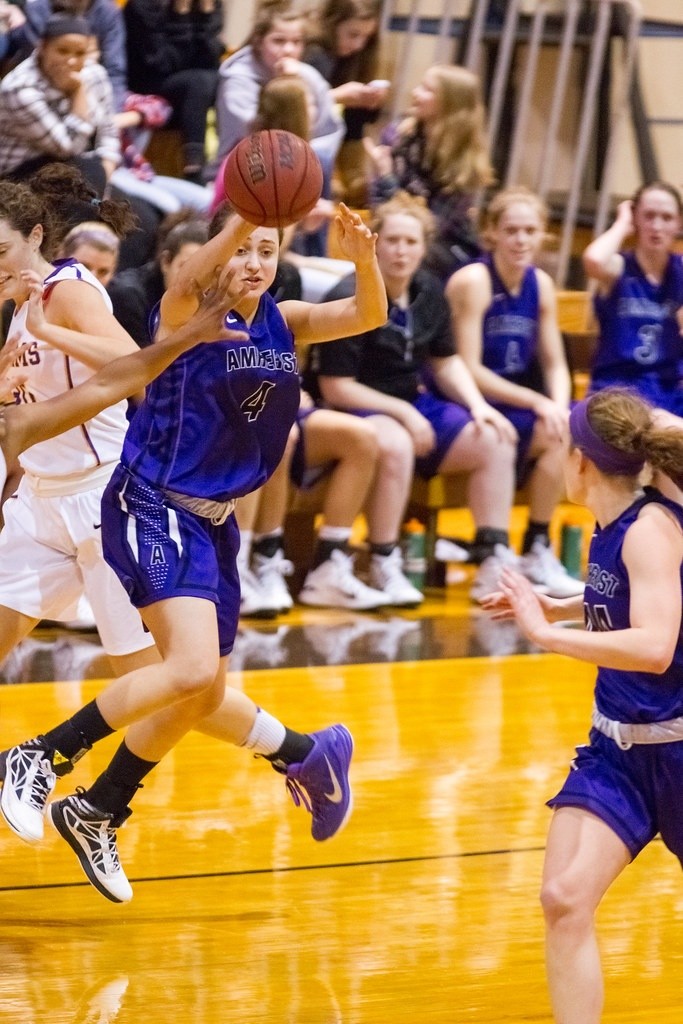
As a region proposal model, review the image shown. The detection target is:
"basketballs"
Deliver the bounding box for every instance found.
[223,129,324,229]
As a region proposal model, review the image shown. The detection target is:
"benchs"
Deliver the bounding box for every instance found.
[288,472,573,586]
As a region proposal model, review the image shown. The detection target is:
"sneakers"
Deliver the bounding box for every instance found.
[48,786,133,903]
[522,544,585,598]
[237,570,275,616]
[0,738,57,843]
[368,546,425,606]
[471,546,519,599]
[253,551,294,612]
[286,724,353,842]
[299,551,391,611]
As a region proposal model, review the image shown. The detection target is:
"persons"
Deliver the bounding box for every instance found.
[0,0,683,619]
[478,386,682,1024]
[0,163,389,902]
